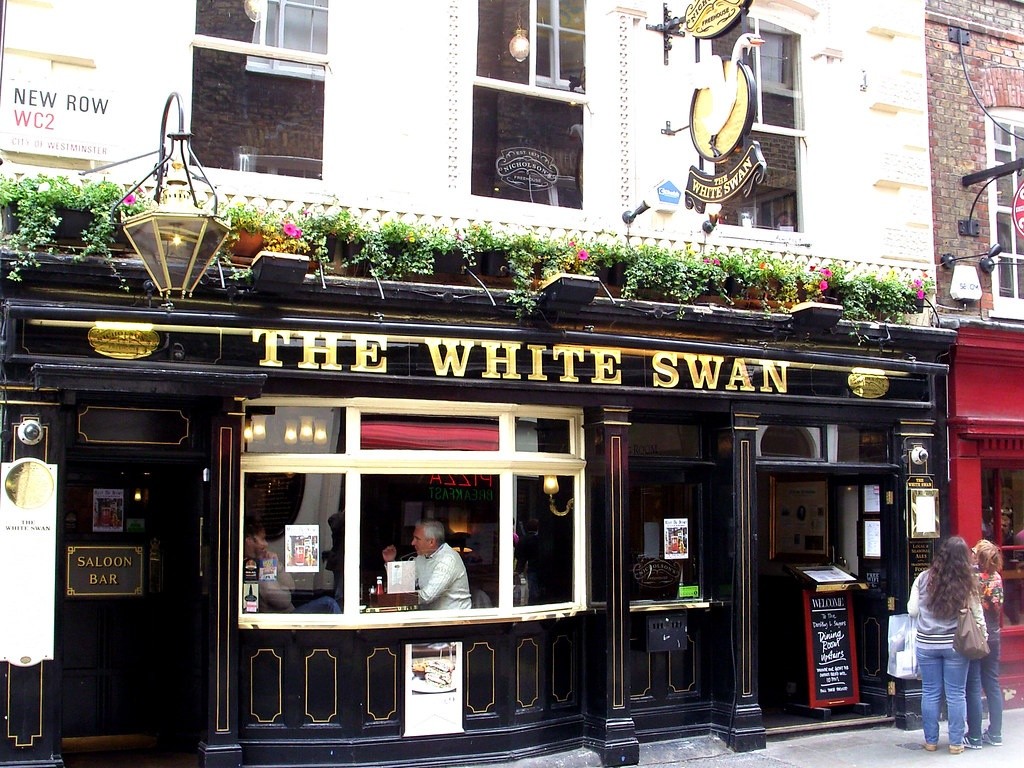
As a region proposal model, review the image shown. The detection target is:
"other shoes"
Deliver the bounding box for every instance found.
[949,744,965,753]
[924,742,937,751]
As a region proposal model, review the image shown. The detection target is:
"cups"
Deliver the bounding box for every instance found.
[1008,561,1020,570]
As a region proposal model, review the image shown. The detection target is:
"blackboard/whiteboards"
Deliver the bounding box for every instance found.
[802,589,860,708]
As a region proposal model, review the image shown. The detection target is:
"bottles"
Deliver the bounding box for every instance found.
[383,582,387,593]
[519,572,529,606]
[513,570,521,606]
[377,576,383,595]
[369,585,376,607]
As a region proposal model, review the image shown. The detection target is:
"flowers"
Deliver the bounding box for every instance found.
[802,261,834,302]
[118,183,146,211]
[698,254,731,285]
[568,237,603,274]
[904,271,936,298]
[281,214,306,242]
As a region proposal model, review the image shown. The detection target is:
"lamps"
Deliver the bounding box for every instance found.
[243,0,264,22]
[790,301,844,330]
[250,251,311,295]
[544,475,574,517]
[79,92,234,299]
[542,273,601,306]
[939,244,1003,267]
[509,0,530,63]
[243,410,327,453]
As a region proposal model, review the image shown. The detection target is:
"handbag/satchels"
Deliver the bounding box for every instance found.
[886,614,922,680]
[953,583,991,660]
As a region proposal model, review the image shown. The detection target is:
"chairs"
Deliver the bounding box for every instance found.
[470,586,492,609]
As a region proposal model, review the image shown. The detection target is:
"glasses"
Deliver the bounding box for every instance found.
[970,547,977,556]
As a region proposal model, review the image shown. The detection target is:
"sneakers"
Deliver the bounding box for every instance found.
[962,734,982,749]
[981,729,1003,746]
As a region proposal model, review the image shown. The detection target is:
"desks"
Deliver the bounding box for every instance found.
[361,591,431,608]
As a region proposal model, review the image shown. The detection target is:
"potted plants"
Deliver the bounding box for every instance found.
[0,172,937,321]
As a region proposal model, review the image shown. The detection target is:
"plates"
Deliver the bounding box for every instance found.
[411,672,455,693]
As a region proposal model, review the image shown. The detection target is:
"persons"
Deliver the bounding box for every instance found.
[962,540,1006,749]
[906,537,988,753]
[983,514,1024,626]
[382,518,473,610]
[242,516,342,613]
